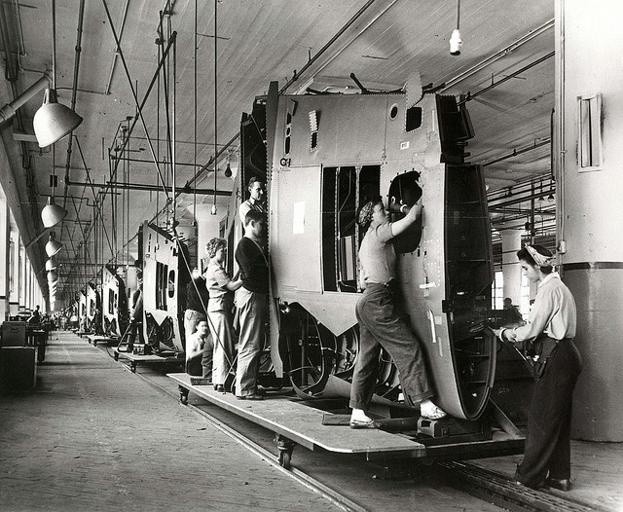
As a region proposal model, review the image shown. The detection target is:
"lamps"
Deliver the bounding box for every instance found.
[0,70,83,147]
[17,195,68,295]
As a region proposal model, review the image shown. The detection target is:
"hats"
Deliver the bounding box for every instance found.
[358,202,373,227]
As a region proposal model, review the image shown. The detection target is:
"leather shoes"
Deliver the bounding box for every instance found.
[214,384,230,392]
[547,479,570,491]
[238,394,263,400]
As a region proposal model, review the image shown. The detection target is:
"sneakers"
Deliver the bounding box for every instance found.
[421,406,447,420]
[350,419,380,428]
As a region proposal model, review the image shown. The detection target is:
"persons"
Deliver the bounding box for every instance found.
[201,333,237,391]
[200,237,244,392]
[227,210,270,401]
[345,176,447,429]
[476,242,585,492]
[182,267,210,372]
[25,311,38,324]
[185,319,207,376]
[39,312,58,325]
[125,289,144,353]
[235,175,267,241]
[34,304,39,319]
